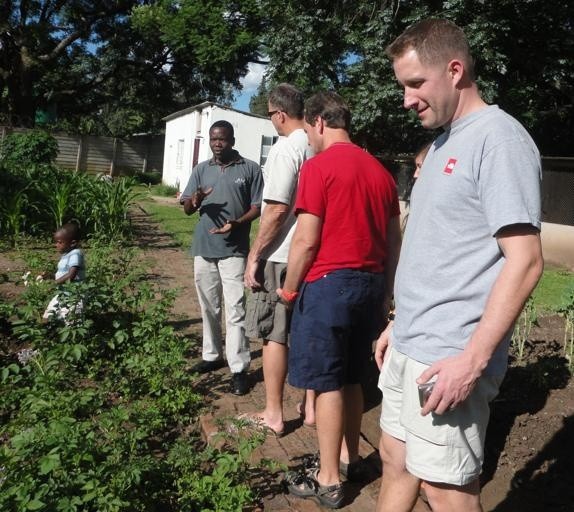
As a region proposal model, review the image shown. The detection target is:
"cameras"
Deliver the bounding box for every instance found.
[417,381,436,408]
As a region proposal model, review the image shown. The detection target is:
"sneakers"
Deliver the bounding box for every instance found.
[315,450,364,481]
[284,468,345,509]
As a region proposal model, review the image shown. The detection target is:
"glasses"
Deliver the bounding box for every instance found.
[266,111,287,120]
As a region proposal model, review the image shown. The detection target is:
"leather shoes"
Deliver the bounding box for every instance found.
[231,371,249,394]
[188,359,224,372]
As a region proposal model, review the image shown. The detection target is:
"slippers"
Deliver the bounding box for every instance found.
[300,414,317,430]
[233,413,284,437]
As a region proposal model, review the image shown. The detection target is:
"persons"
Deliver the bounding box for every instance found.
[275,90,402,511]
[234,80,316,437]
[411,143,431,180]
[177,120,265,396]
[37,223,85,327]
[373,17,545,512]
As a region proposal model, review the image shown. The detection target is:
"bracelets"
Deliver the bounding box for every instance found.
[386,313,396,321]
[240,216,246,223]
[275,286,299,302]
